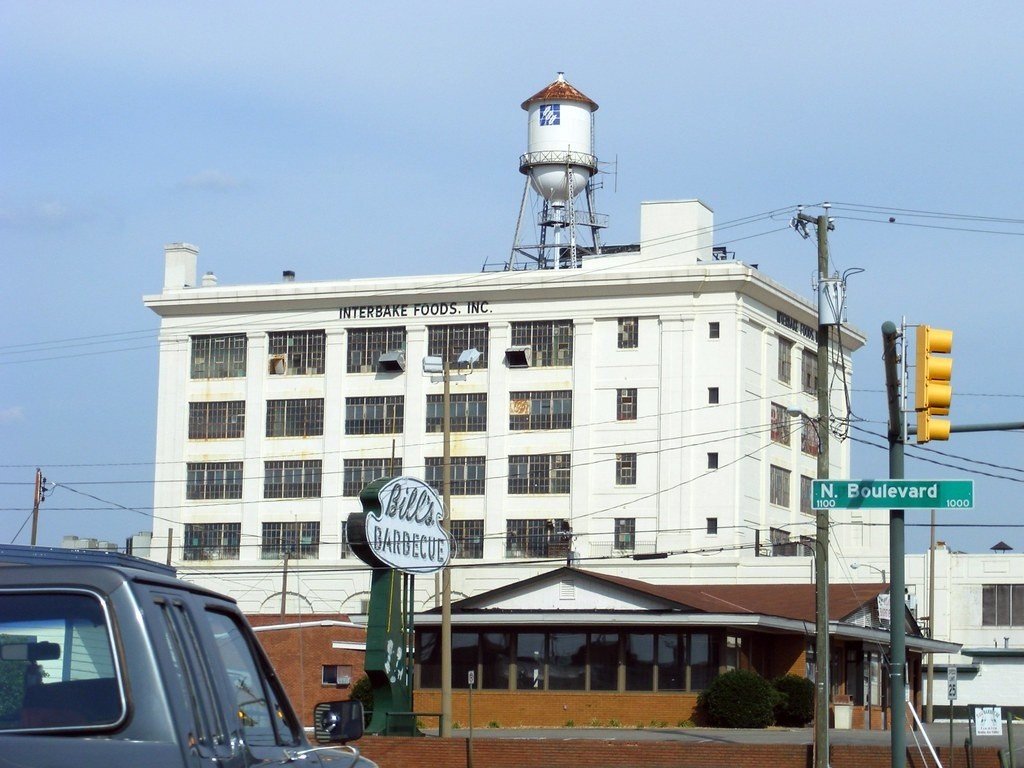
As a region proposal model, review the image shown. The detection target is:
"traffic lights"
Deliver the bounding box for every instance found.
[917,410,951,445]
[915,324,953,416]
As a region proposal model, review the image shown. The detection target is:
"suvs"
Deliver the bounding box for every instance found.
[0,542,377,768]
